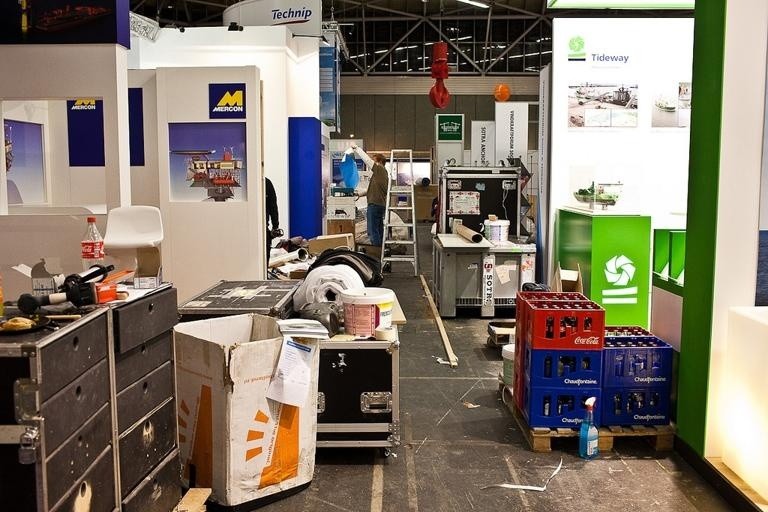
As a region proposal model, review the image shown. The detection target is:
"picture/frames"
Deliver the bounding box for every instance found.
[3,116,51,206]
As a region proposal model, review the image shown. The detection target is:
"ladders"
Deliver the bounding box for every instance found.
[380,149,421,277]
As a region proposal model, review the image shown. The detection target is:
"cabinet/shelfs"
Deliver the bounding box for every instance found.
[317,324,400,458]
[555,207,652,333]
[0,278,182,511]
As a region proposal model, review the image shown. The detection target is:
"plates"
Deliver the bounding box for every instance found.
[0,312,51,335]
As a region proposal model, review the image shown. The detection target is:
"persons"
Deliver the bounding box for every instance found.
[265,179,282,268]
[350,143,389,247]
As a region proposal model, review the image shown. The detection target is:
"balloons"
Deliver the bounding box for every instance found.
[494,84,510,102]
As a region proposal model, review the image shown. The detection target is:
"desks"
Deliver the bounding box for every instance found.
[414,184,438,221]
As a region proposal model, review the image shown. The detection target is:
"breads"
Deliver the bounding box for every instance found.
[2,317,35,330]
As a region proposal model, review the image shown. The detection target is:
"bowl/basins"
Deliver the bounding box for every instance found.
[484,220,510,245]
[573,192,616,205]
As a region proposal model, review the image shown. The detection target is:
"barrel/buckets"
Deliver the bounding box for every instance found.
[484,219,510,241]
[501,344,515,384]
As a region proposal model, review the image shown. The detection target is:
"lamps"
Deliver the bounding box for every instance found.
[459,0,493,8]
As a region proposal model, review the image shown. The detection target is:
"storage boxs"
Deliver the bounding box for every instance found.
[356,243,381,259]
[308,235,355,256]
[173,311,321,506]
[326,194,356,220]
[326,219,355,242]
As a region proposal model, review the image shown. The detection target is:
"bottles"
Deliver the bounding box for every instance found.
[80,216,104,275]
[514,291,671,426]
[64,265,114,290]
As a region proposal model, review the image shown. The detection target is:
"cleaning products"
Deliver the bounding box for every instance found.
[579,396,599,459]
